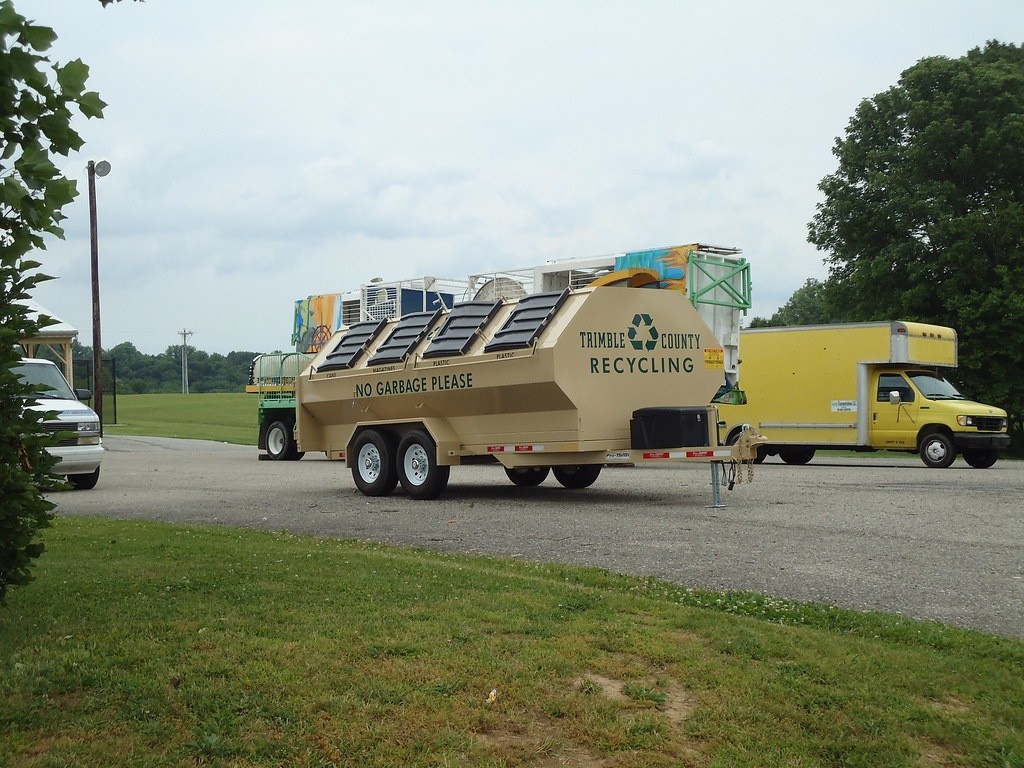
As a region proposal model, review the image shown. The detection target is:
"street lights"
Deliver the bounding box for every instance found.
[86,159,112,437]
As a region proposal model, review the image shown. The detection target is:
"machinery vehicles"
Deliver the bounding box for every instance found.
[244,242,753,461]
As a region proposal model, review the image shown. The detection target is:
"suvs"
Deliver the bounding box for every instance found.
[8,359,104,490]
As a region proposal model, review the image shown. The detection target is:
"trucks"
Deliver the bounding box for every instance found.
[710,319,1009,470]
[294,286,770,500]
